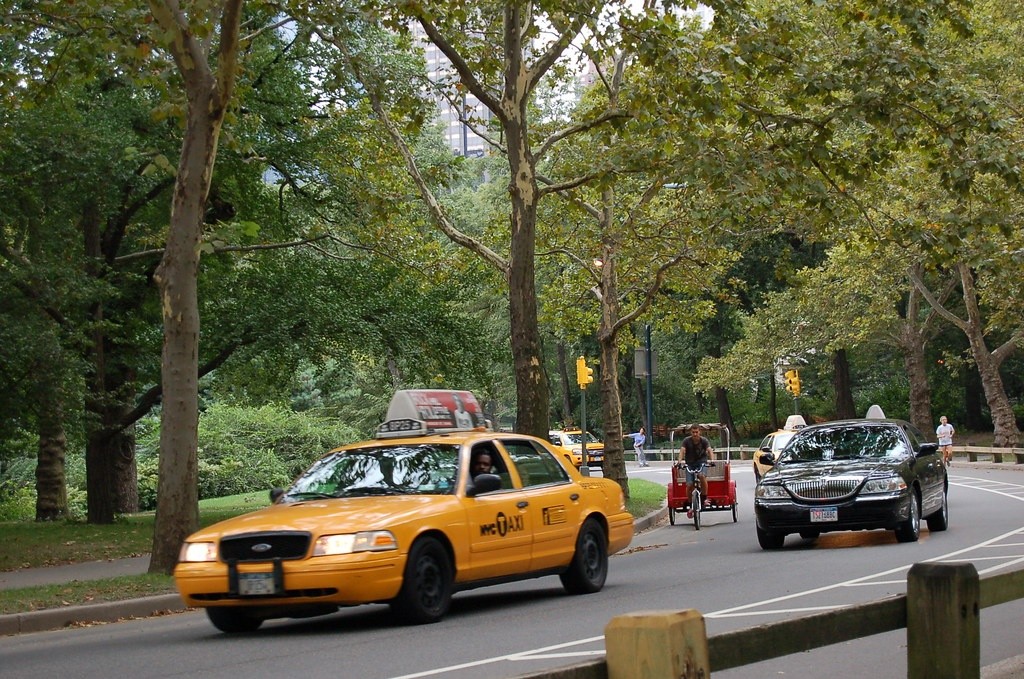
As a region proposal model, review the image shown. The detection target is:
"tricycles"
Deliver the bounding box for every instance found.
[667,422,739,530]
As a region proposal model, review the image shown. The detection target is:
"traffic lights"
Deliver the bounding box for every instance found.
[585,367,593,383]
[785,369,795,393]
[789,377,800,392]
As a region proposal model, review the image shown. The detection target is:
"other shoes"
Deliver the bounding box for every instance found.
[639,464,644,467]
[644,463,648,465]
[947,461,950,466]
[687,509,692,518]
[703,499,711,505]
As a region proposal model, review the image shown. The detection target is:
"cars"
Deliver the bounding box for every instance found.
[548,426,605,470]
[753,420,948,551]
[753,414,808,485]
[175,388,635,636]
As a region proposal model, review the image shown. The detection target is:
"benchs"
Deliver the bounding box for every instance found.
[672,461,730,482]
[520,473,552,486]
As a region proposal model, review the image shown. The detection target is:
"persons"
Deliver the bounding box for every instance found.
[678,422,717,519]
[623,426,649,467]
[936,416,955,467]
[463,448,501,493]
